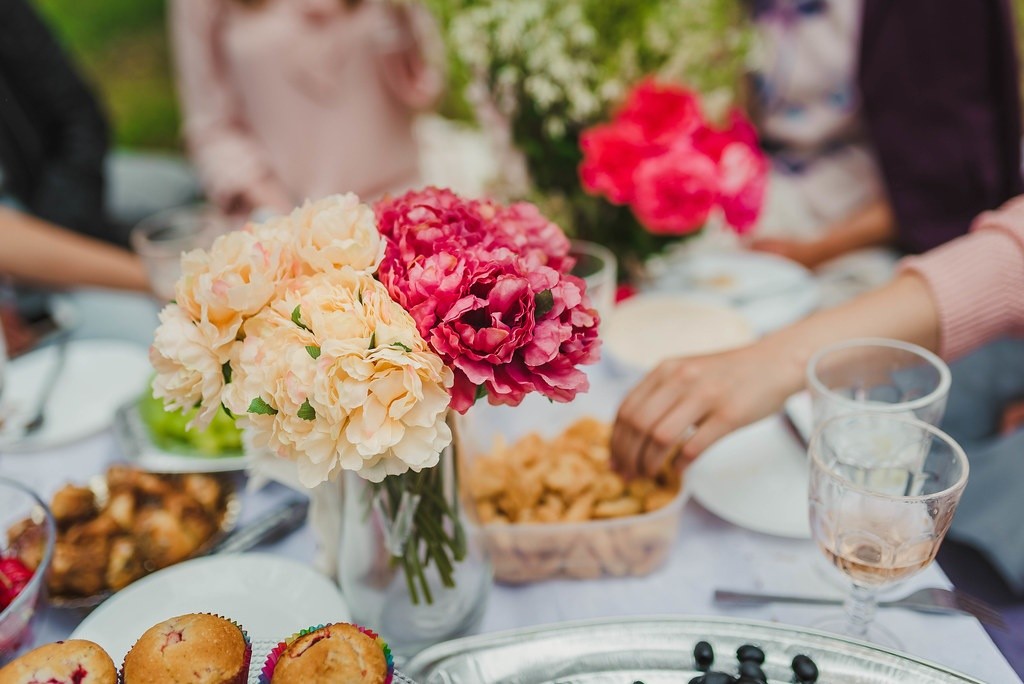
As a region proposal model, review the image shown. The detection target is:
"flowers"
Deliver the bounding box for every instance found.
[452,0,764,226]
[150,183,590,482]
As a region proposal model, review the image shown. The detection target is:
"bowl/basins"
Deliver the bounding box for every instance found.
[478,468,691,586]
[0,474,55,663]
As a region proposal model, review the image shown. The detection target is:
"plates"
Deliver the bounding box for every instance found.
[1,343,138,447]
[57,474,310,608]
[65,555,349,684]
[117,407,257,473]
[607,290,755,364]
[692,409,812,537]
[394,615,981,684]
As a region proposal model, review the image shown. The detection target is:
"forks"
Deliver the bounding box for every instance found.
[715,587,1009,631]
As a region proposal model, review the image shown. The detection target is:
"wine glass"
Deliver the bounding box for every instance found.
[807,409,970,642]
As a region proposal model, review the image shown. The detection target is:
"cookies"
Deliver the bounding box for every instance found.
[458,416,681,584]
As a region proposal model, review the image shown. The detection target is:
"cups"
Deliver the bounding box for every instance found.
[571,238,618,327]
[135,205,225,304]
[806,338,951,430]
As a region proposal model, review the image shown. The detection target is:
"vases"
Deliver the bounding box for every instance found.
[572,189,703,258]
[303,397,491,637]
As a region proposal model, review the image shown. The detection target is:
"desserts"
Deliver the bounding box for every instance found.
[0,611,394,684]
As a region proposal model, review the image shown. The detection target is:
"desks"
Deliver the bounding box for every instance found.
[1,165,1024,684]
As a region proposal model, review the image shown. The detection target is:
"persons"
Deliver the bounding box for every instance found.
[168,0,444,233]
[606,1,1023,604]
[0,0,190,359]
